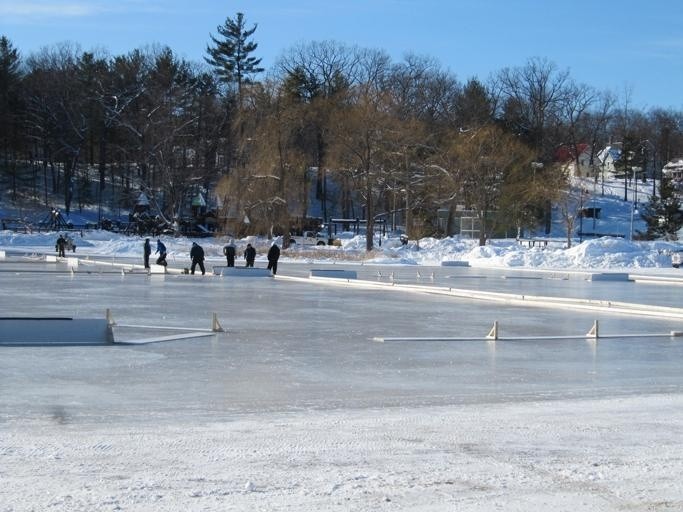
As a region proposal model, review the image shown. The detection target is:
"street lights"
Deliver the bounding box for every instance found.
[530,161,543,181]
[617,148,634,201]
[639,146,657,198]
[631,165,643,212]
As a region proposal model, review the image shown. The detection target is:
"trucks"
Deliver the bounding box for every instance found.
[669,251,682,268]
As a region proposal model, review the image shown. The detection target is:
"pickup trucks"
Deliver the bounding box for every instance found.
[288,230,327,246]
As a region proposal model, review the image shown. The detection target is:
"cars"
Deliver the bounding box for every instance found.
[179,222,232,238]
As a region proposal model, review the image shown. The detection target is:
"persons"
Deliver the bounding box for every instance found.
[144,239,279,275]
[56,235,66,257]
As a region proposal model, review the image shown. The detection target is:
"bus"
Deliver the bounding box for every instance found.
[285,214,324,236]
